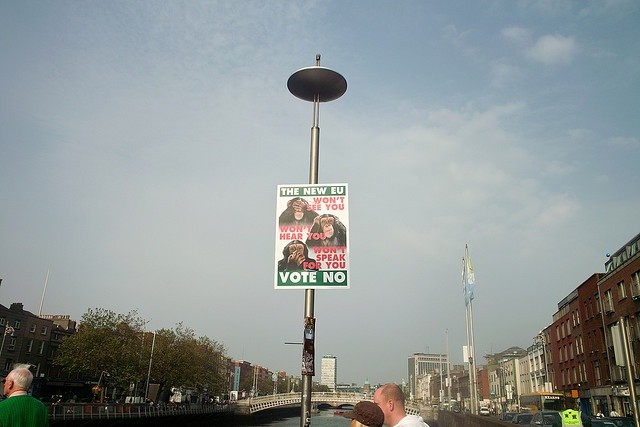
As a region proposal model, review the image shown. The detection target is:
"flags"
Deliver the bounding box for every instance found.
[461,260,469,306]
[466,247,475,303]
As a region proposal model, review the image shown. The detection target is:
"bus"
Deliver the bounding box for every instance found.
[519,392,566,415]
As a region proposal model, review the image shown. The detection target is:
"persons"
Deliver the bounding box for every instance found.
[627,410,633,417]
[596,410,604,419]
[0,367,49,427]
[610,409,620,417]
[373,383,430,427]
[552,397,591,427]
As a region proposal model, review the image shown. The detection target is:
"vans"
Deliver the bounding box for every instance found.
[480,407,489,416]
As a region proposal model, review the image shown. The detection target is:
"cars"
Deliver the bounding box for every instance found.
[604,417,635,427]
[530,412,562,427]
[512,413,533,425]
[589,420,618,427]
[504,413,517,421]
